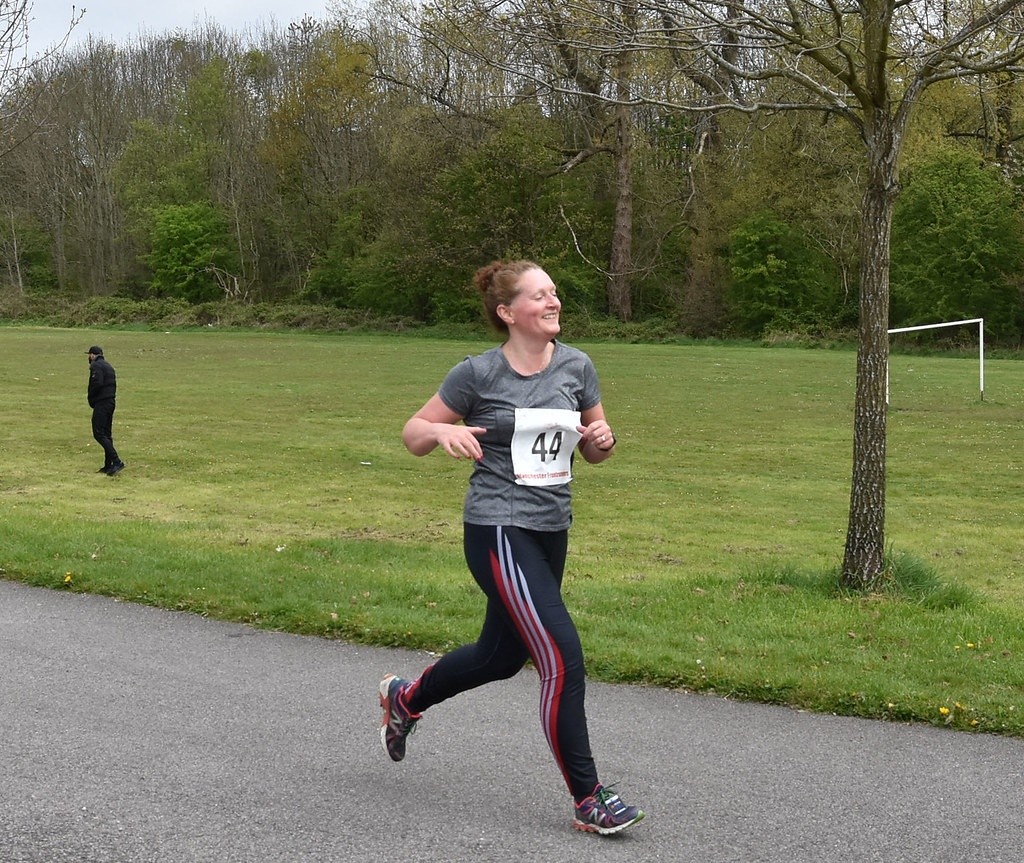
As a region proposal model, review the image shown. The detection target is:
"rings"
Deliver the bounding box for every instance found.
[601,435,605,442]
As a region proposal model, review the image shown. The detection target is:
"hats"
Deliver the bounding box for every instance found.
[84,346,104,354]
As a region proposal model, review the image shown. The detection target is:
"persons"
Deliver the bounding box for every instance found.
[84,346,124,477]
[377,258,646,835]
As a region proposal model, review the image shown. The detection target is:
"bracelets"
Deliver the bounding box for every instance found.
[595,436,616,451]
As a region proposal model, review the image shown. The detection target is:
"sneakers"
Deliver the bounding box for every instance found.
[379,668,422,764]
[97,460,125,476]
[574,785,646,837]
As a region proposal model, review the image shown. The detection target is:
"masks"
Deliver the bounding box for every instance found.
[88,356,93,364]
